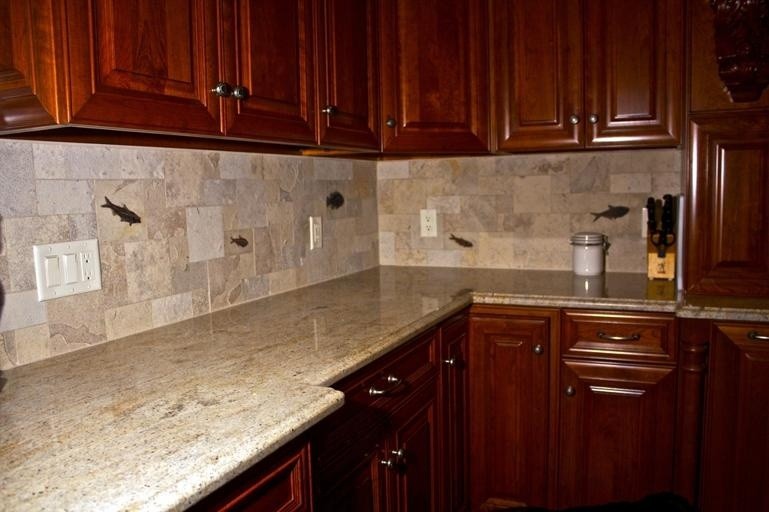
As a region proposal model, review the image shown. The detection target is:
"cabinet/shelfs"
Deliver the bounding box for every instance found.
[557,308,680,512]
[0,0,318,156]
[375,0,490,157]
[442,309,468,512]
[468,304,561,512]
[696,320,769,512]
[684,111,769,299]
[186,440,312,512]
[315,0,381,152]
[309,326,444,512]
[490,0,691,152]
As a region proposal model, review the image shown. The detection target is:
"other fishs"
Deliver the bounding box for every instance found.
[448,233,473,247]
[100,195,141,227]
[326,191,345,210]
[590,204,630,222]
[229,235,248,248]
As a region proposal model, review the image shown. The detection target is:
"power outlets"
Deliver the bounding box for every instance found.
[33,238,101,301]
[420,208,437,238]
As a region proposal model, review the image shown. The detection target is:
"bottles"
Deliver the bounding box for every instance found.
[571,232,607,276]
[572,276,606,298]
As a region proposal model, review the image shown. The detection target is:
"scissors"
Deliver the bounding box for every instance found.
[650,229,676,257]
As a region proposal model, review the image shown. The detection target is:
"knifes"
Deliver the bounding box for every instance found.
[645,193,677,234]
